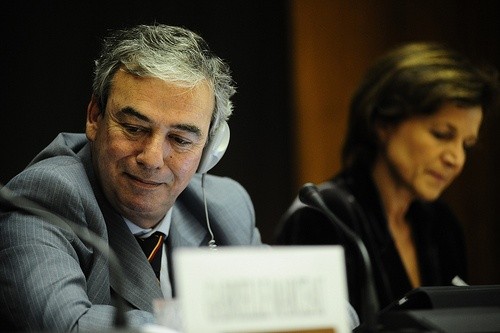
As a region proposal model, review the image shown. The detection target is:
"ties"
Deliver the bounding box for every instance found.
[133,232,164,281]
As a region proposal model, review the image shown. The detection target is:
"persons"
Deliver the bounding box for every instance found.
[274,42,500,333]
[0,25,360,333]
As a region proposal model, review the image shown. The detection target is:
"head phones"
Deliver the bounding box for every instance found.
[196,120,230,174]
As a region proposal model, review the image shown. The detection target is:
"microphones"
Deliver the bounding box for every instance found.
[300,183,382,333]
